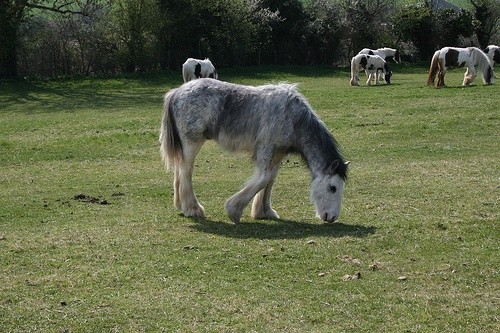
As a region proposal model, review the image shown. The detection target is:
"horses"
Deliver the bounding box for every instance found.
[157,77,351,225]
[350,54,394,86]
[427,47,495,88]
[463,45,500,85]
[357,45,403,80]
[183,58,218,85]
